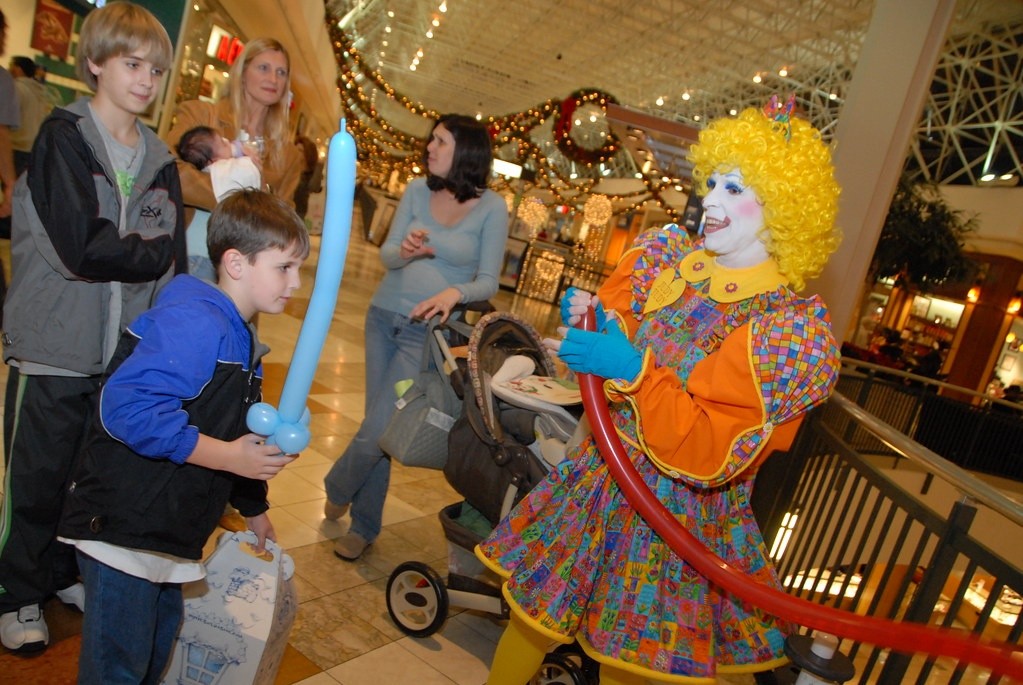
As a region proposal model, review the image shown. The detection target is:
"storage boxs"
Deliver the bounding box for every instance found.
[164,530,297,685]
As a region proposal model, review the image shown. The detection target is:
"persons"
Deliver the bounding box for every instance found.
[868,327,1023,416]
[323,113,508,561]
[537,227,585,250]
[164,37,318,533]
[474,90,842,685]
[0,0,189,653]
[54,183,310,685]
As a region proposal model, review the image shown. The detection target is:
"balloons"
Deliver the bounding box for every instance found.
[246,117,356,456]
[574,294,1023,685]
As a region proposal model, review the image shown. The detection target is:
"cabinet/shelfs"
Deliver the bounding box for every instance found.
[902,313,955,357]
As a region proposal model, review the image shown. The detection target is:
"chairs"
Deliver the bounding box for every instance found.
[905,365,952,394]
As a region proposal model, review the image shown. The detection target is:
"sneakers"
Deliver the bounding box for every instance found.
[0,603,50,653]
[57,581,85,613]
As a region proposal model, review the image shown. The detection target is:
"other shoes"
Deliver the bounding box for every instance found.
[324,499,348,520]
[334,531,374,561]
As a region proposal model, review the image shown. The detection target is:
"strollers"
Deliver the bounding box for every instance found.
[388,299,600,685]
